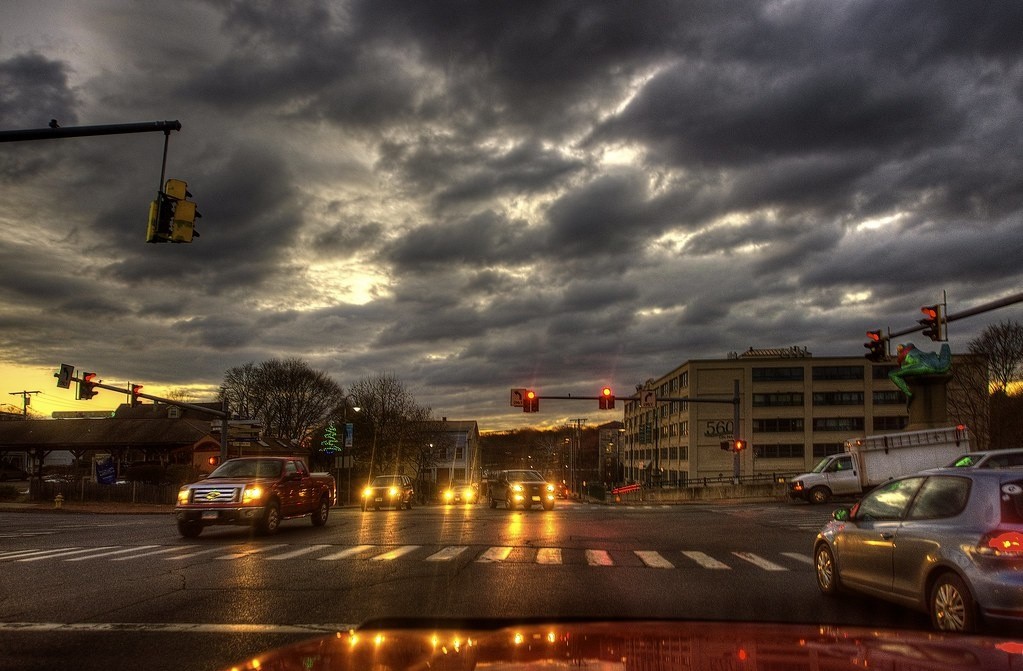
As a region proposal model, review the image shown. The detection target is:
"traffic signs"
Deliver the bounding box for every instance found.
[640,390,655,408]
[510,388,527,407]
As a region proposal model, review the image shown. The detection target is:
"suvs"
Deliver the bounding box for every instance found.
[362,474,415,511]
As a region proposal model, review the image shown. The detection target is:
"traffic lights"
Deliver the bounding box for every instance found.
[81,372,98,399]
[918,305,943,342]
[601,387,612,396]
[130,383,143,410]
[720,441,729,450]
[608,396,616,409]
[523,398,531,413]
[531,398,539,412]
[173,200,202,244]
[208,456,219,466]
[526,391,535,399]
[864,329,887,364]
[166,179,192,201]
[598,396,607,410]
[736,440,746,450]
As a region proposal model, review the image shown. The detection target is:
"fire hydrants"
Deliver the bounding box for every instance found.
[54,493,66,511]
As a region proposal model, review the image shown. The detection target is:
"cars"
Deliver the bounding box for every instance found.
[812,465,1023,637]
[920,449,1023,474]
[448,486,477,505]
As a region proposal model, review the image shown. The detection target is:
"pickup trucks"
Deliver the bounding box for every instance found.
[175,456,336,541]
[487,469,553,512]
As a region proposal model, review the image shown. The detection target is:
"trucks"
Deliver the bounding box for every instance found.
[786,426,972,506]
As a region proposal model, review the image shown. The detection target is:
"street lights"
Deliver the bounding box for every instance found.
[338,392,360,505]
